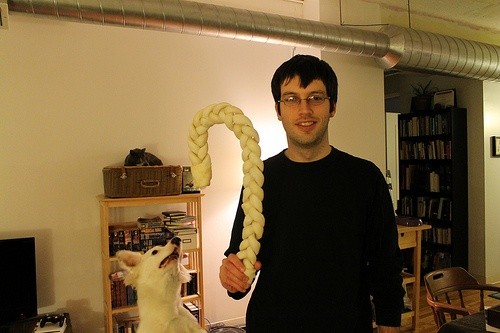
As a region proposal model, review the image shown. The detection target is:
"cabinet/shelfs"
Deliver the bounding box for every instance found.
[398,106,470,284]
[372,223,432,333]
[96,193,204,333]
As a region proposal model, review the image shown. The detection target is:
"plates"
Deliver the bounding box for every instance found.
[210,326,246,333]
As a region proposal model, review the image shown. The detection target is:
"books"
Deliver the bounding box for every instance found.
[399,139,451,160]
[399,113,450,137]
[109,210,198,257]
[422,220,452,244]
[184,302,200,322]
[402,196,450,219]
[111,251,199,308]
[112,316,139,333]
[401,163,447,193]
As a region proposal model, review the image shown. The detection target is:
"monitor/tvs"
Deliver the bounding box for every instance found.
[0,235,37,324]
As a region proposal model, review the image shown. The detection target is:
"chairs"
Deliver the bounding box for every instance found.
[424,266,500,328]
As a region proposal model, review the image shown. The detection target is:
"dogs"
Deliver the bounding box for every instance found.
[115,237,209,333]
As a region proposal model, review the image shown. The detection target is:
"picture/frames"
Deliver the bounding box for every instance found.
[492,136,500,156]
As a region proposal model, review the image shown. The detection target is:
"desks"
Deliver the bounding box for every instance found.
[438,310,494,333]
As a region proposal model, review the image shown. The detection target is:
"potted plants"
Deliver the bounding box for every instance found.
[409,79,440,112]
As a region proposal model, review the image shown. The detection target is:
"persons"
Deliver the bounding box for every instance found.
[218,54,406,332]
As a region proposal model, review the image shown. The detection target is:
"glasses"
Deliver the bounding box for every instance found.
[276,94,331,105]
